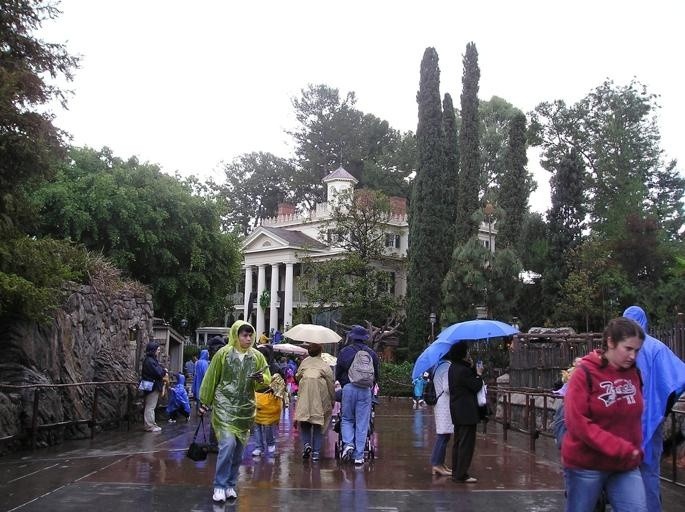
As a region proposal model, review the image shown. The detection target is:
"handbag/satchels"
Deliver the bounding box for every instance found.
[476,383,491,407]
[184,440,208,462]
[137,379,154,392]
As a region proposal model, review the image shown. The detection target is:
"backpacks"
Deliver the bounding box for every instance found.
[348,343,376,389]
[551,363,644,450]
[422,360,449,406]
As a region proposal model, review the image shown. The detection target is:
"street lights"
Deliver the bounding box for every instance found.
[179,316,188,338]
[511,316,521,332]
[429,311,436,345]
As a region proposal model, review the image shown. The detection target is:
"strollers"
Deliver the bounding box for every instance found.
[335,387,378,460]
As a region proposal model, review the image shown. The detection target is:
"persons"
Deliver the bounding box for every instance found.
[142,320,379,502]
[552,306,685,512]
[412,340,487,481]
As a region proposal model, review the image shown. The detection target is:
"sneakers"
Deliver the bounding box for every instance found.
[353,455,366,465]
[300,444,310,458]
[210,484,226,504]
[312,451,320,461]
[266,443,278,456]
[226,487,238,500]
[430,463,479,483]
[251,443,263,455]
[144,414,191,432]
[341,443,354,462]
